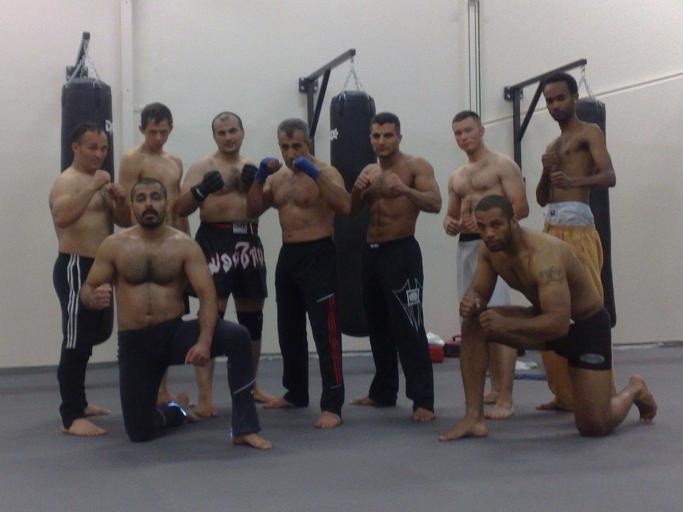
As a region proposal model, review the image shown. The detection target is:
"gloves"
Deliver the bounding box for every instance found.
[291,156,321,179]
[256,156,283,184]
[190,169,224,202]
[240,162,259,185]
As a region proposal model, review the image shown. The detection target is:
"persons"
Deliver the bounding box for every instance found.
[350,111,442,421]
[47,120,130,438]
[118,101,192,404]
[443,109,529,420]
[244,118,353,430]
[173,110,275,418]
[437,194,657,443]
[78,177,273,451]
[533,71,615,410]
